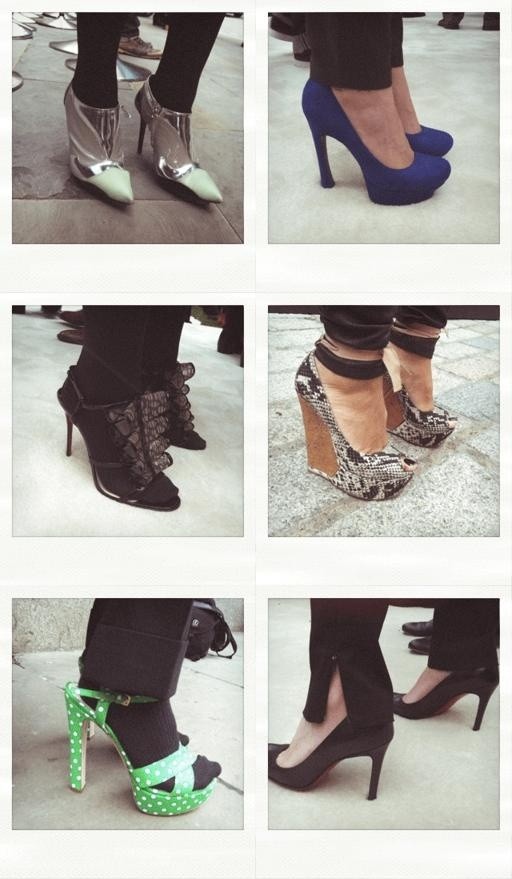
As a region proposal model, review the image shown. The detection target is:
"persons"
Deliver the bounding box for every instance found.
[119,13,163,60]
[58,306,87,345]
[404,621,435,655]
[295,308,457,501]
[269,14,313,68]
[268,597,499,801]
[440,13,499,29]
[216,307,243,367]
[302,13,452,205]
[64,13,224,205]
[67,599,221,816]
[57,306,206,509]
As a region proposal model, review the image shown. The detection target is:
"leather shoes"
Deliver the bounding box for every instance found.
[118,37,161,60]
[57,310,87,345]
[402,621,434,654]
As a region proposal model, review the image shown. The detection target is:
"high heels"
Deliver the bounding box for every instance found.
[58,362,205,511]
[64,79,134,204]
[383,327,457,446]
[134,74,223,203]
[392,667,499,730]
[65,681,218,816]
[302,79,453,206]
[294,339,413,500]
[268,715,393,799]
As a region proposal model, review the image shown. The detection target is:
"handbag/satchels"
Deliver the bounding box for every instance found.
[184,598,237,661]
